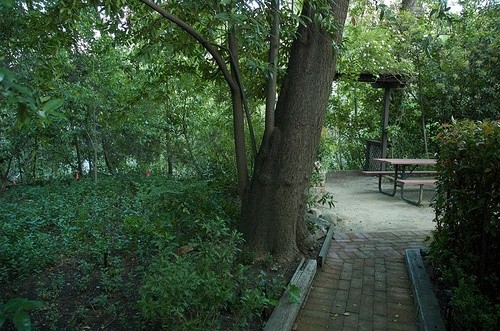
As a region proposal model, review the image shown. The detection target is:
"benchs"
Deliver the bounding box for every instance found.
[362,170,442,193]
[396,179,439,205]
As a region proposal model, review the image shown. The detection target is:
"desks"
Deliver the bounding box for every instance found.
[373,157,448,196]
